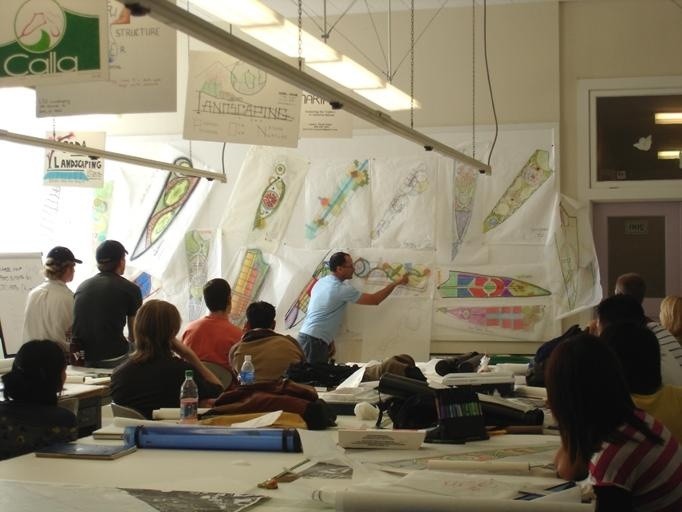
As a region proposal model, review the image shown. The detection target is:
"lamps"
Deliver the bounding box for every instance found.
[656,149,681,160]
[654,112,682,126]
[192,0,421,111]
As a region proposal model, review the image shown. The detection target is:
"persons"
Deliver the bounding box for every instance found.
[298,252,409,365]
[180,279,242,380]
[590,273,682,389]
[228,302,304,389]
[110,299,223,420]
[22,246,82,363]
[0,339,79,460]
[70,240,143,369]
[544,335,682,511]
[554,322,682,480]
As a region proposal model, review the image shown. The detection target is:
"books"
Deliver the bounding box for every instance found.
[91,423,125,440]
[34,441,137,460]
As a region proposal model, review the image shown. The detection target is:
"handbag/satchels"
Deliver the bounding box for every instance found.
[434,388,489,442]
[361,354,415,381]
[526,324,589,387]
[386,390,438,430]
[287,359,358,387]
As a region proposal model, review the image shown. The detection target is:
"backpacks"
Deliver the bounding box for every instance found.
[202,374,337,429]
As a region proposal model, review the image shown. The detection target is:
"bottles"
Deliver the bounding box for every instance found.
[240,355,256,386]
[178,370,199,425]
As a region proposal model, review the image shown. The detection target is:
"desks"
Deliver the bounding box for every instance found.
[1,362,603,512]
[0,364,115,409]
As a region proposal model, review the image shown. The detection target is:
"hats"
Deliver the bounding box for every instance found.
[95,240,128,264]
[47,247,82,263]
